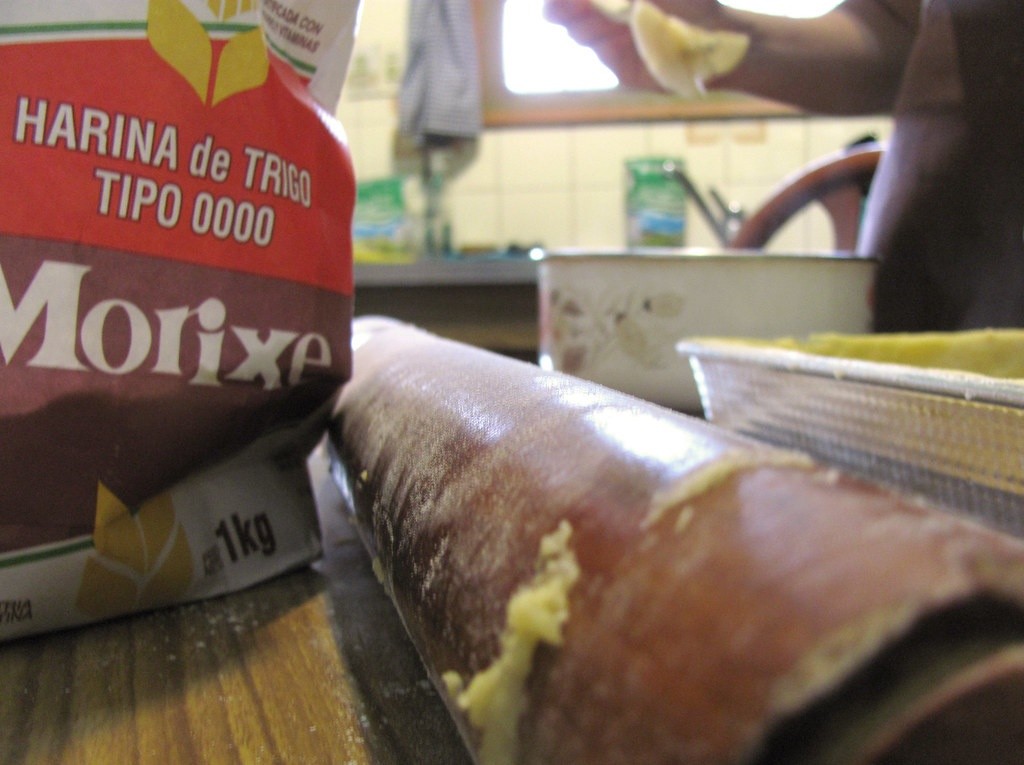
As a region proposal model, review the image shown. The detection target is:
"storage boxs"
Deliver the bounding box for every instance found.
[676,338,1024,540]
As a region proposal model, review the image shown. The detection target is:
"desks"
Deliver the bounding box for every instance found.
[353,264,542,347]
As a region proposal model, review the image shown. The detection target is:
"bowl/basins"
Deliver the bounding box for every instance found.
[529,248,877,416]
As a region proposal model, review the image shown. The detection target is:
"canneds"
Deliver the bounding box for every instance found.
[353,175,414,255]
[623,153,688,247]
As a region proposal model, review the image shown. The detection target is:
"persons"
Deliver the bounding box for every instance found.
[541,0,1024,334]
